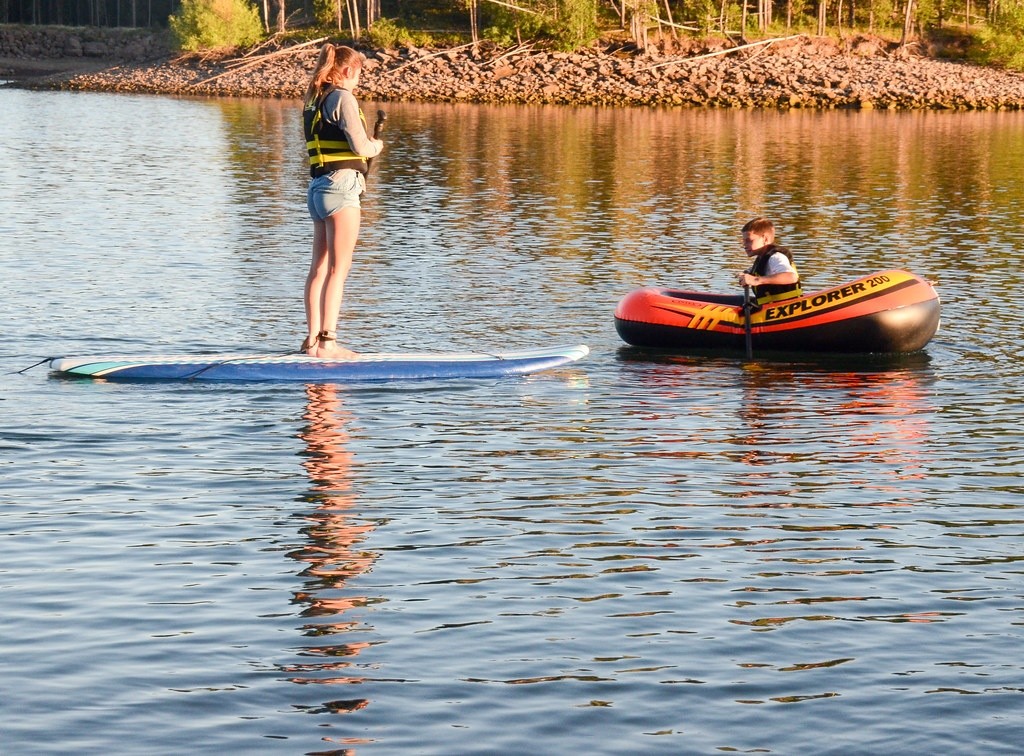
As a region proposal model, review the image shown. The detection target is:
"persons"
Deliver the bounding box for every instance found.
[735,218,803,305]
[302,43,384,358]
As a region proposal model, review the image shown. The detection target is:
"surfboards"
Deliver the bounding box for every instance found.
[48,341,594,381]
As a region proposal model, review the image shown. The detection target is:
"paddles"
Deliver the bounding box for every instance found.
[740,269,754,364]
[359,108,388,205]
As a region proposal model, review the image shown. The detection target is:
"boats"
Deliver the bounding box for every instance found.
[614,270,941,352]
